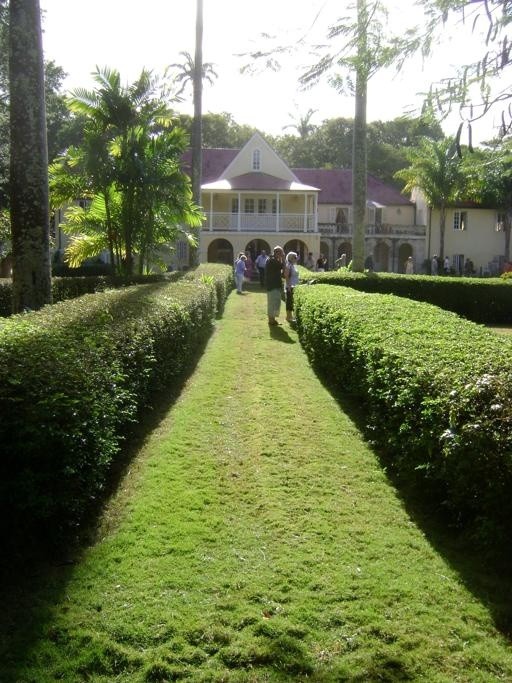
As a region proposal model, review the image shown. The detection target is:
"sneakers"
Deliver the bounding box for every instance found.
[286,316,297,322]
[268,320,283,326]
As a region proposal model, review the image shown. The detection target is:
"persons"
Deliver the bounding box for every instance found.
[304,253,316,272]
[336,254,347,269]
[316,254,327,272]
[465,258,476,274]
[443,256,449,275]
[404,256,414,274]
[365,253,375,272]
[430,254,438,275]
[234,245,301,326]
